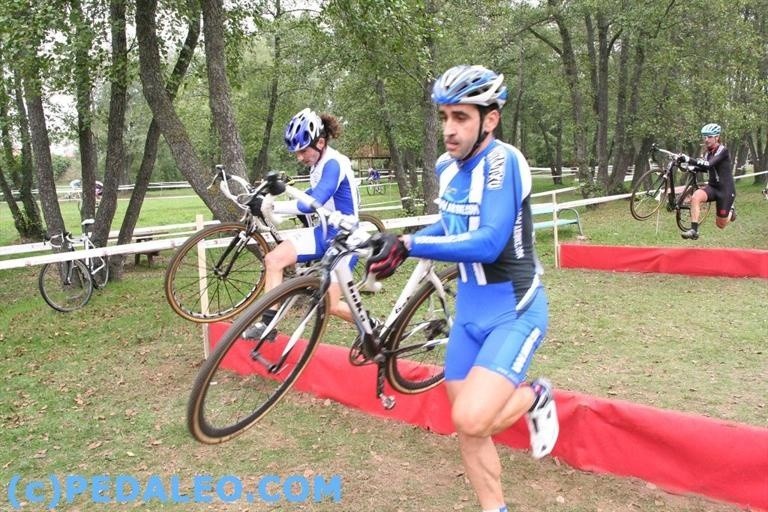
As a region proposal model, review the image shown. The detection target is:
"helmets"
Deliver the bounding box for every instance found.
[700,122,724,137]
[429,62,510,112]
[284,106,328,154]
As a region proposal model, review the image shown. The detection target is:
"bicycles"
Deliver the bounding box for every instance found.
[39,219,109,312]
[165,169,462,446]
[366,178,385,195]
[630,143,712,231]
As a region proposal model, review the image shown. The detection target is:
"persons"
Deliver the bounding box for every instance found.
[94,185,102,200]
[365,167,382,193]
[676,123,737,241]
[241,108,381,351]
[355,64,560,512]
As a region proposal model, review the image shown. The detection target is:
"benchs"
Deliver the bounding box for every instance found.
[532,207,584,242]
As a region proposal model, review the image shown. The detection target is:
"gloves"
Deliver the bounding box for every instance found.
[364,235,409,279]
[246,197,267,218]
[676,155,685,165]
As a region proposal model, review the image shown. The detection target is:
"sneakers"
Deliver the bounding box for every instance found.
[681,228,700,241]
[368,317,385,332]
[525,376,561,460]
[730,206,737,222]
[240,321,278,343]
